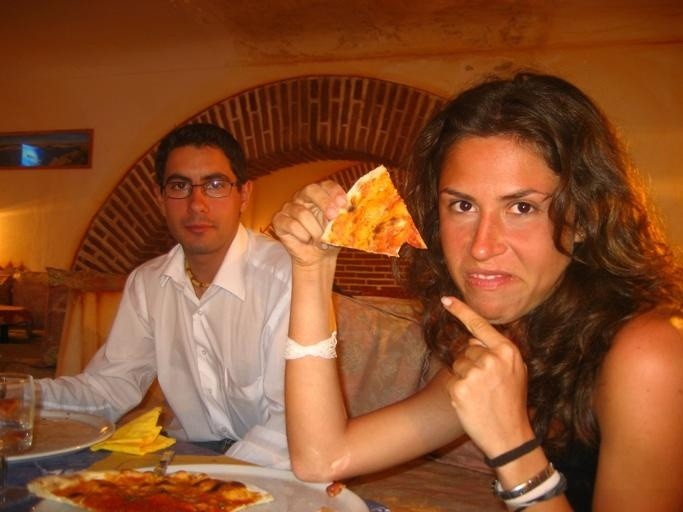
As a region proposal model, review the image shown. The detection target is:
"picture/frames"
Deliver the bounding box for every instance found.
[0,128,94,169]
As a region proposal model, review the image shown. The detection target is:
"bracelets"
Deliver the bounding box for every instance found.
[483,436,544,467]
[280,328,338,360]
[488,461,569,510]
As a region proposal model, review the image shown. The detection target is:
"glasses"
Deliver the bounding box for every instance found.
[161,179,238,198]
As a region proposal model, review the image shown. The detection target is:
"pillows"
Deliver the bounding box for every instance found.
[332,292,430,418]
[353,296,495,476]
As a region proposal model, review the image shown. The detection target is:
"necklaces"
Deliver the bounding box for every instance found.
[180,252,214,294]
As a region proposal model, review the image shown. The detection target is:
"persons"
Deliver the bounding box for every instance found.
[0,122,292,470]
[270,68,681,511]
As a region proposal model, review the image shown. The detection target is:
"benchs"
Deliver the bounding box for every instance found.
[46,261,521,512]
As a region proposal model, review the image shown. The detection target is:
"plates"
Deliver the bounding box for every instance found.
[0,409,116,464]
[30,462,370,512]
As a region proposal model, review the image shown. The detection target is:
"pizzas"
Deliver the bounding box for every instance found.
[24,468,275,511]
[319,163,428,260]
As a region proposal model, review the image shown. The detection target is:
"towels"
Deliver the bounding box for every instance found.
[91,406,176,456]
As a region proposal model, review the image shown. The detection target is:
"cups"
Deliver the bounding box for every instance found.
[0,372,36,455]
